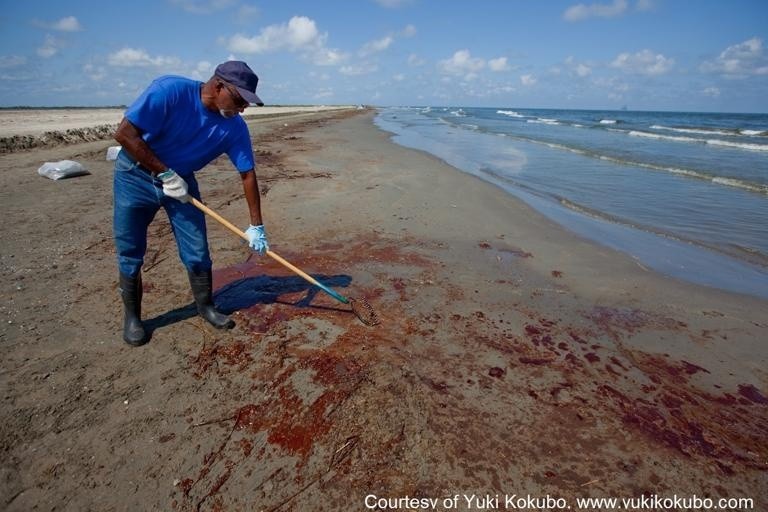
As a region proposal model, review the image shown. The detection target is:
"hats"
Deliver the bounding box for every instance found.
[214,61,263,107]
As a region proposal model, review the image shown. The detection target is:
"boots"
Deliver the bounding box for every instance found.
[187,271,234,330]
[119,273,147,346]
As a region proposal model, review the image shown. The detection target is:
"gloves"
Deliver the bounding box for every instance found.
[240,225,269,255]
[158,170,189,203]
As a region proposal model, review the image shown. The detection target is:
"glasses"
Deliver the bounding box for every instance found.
[223,82,244,107]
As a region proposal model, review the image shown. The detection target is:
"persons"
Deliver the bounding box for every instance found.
[110,59,271,347]
